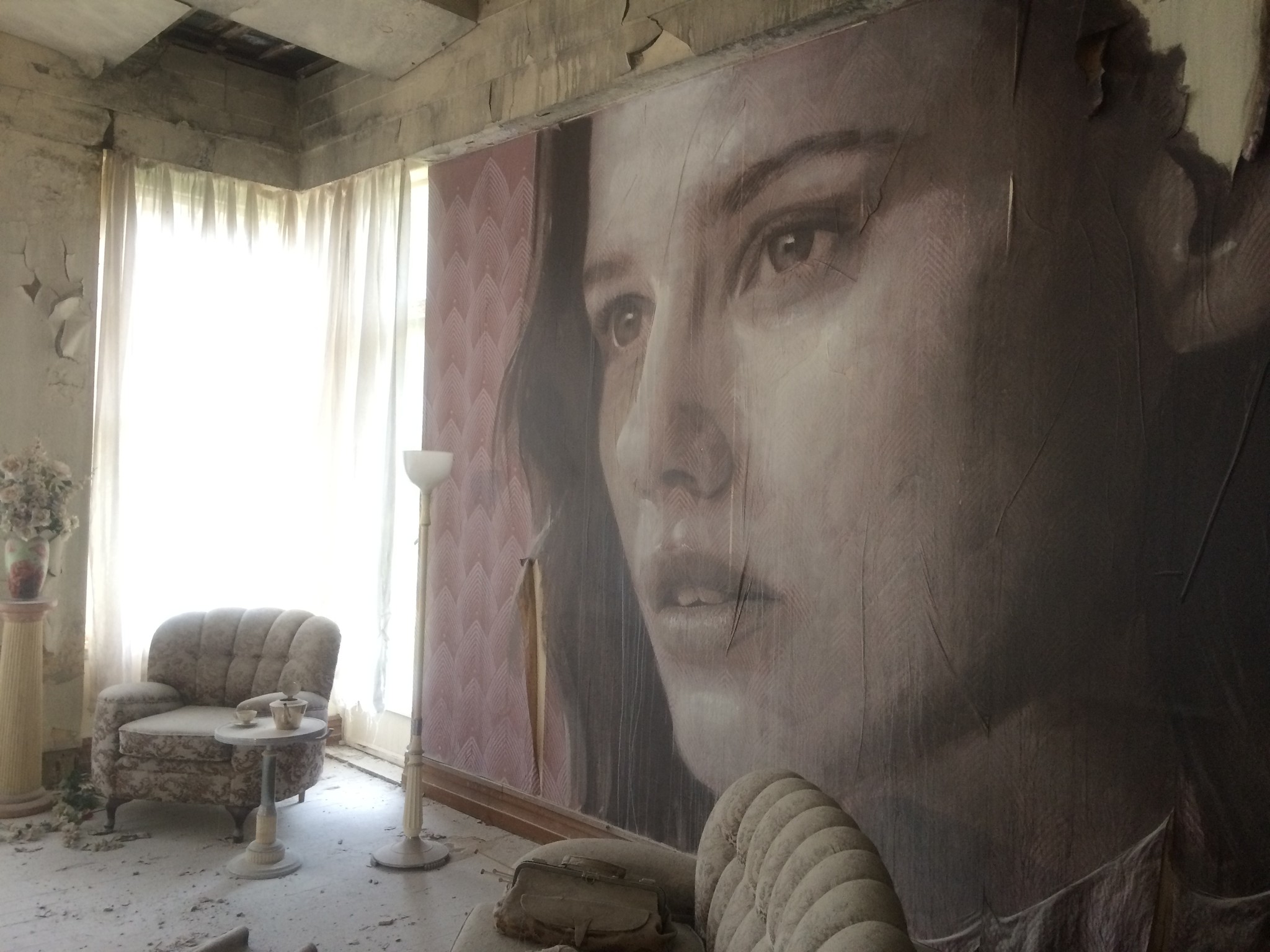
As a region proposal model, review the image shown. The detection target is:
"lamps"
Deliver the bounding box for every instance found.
[372,452,452,870]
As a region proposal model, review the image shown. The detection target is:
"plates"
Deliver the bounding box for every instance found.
[233,721,256,726]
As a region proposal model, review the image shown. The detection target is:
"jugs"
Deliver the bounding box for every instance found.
[269,679,308,730]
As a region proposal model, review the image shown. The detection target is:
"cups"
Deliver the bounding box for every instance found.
[236,710,257,724]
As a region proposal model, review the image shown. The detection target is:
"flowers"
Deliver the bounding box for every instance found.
[0,444,80,542]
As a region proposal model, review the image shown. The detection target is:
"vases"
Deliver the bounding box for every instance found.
[5,534,50,601]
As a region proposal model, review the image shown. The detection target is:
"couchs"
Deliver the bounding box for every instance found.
[447,768,911,952]
[93,606,338,841]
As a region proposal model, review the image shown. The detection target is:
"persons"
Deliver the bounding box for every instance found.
[503,1,1270,952]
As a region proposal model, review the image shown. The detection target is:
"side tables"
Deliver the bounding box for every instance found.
[212,720,325,880]
